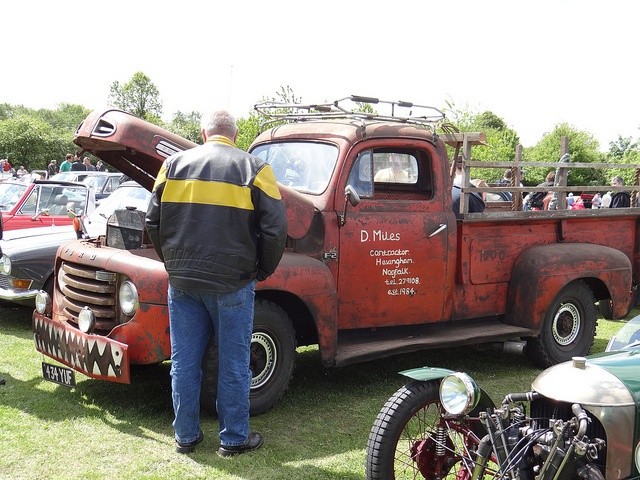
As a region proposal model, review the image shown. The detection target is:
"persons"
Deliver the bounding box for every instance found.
[496,169,525,198]
[568,192,575,203]
[601,190,613,209]
[591,192,601,208]
[0,159,12,175]
[523,171,567,210]
[145,111,287,457]
[48,159,58,175]
[609,177,630,208]
[40,174,49,180]
[17,166,28,178]
[373,153,416,184]
[450,155,489,213]
[58,154,109,173]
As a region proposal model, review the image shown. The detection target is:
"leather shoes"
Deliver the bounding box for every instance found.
[219,432,264,457]
[176,430,204,453]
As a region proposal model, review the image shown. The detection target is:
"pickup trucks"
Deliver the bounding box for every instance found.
[34,95,640,417]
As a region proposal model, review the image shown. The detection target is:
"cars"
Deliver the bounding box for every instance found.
[80,173,124,202]
[0,181,151,307]
[49,172,104,189]
[0,180,98,231]
